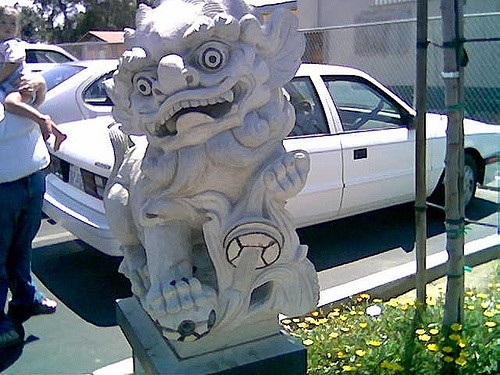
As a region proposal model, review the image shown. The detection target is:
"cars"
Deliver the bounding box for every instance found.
[36,63,500,257]
[34,59,123,124]
[22,45,76,74]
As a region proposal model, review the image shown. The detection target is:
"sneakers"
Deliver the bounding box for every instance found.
[0,327,20,347]
[11,296,57,314]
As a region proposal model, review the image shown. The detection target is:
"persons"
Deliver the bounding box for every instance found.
[0,62,59,352]
[1,6,68,150]
